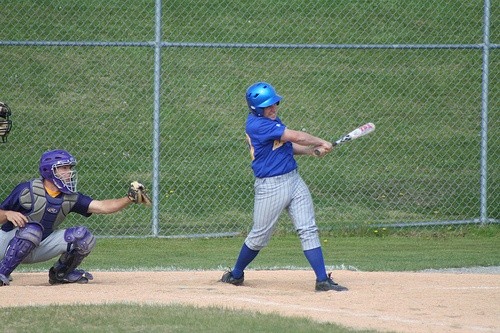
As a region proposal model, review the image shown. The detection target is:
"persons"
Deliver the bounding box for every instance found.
[0,150,152,286]
[217,82,350,292]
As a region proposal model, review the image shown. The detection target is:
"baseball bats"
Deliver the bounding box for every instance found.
[313,122,375,155]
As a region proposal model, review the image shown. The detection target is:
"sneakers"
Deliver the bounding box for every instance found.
[218,266,244,287]
[315,272,348,292]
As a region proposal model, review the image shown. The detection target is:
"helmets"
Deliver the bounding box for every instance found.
[40,150,77,178]
[246,81,282,118]
[0,101,11,117]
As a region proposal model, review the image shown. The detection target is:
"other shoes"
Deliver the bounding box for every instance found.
[49,267,88,284]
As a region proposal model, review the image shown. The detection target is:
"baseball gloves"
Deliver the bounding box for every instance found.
[128,181,152,205]
